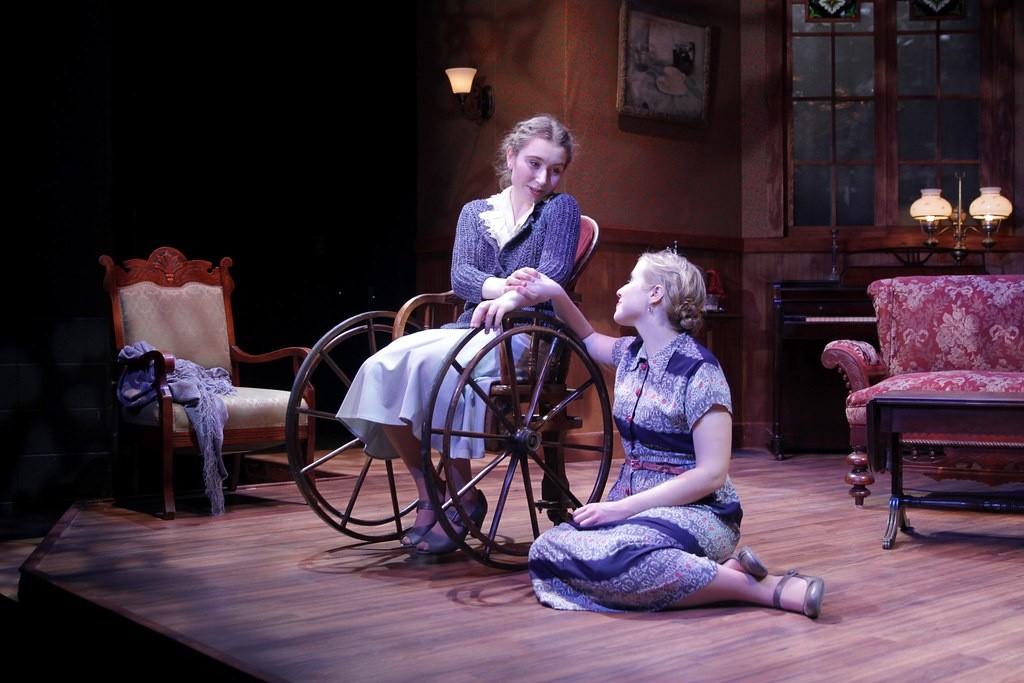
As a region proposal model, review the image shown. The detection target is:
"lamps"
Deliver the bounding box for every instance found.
[445,67,496,125]
[910,171,1013,260]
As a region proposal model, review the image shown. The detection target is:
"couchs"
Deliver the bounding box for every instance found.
[821,275,1024,508]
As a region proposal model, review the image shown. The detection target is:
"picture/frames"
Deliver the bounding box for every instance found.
[614,0,718,144]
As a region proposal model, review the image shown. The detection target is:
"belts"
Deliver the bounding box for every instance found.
[625,458,687,474]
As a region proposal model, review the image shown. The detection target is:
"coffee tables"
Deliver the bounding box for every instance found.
[866,390,1024,550]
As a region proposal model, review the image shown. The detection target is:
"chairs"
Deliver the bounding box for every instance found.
[98,246,315,519]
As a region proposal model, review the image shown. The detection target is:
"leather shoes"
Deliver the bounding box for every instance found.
[772,569,824,619]
[724,545,768,580]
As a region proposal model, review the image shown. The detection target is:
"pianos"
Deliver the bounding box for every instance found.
[764,262,992,463]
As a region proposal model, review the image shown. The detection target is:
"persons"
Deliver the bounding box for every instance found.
[334,113,580,557]
[511,247,826,621]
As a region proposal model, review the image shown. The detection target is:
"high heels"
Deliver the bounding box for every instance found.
[416,489,486,553]
[400,480,450,547]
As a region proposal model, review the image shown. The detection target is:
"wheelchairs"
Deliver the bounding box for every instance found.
[280,211,616,572]
